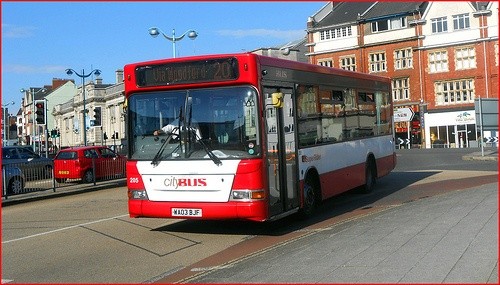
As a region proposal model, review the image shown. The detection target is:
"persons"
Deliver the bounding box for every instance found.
[154,104,203,141]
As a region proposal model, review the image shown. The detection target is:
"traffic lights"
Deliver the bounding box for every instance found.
[94,107,102,128]
[34,100,46,125]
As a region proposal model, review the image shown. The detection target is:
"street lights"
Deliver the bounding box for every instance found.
[148,27,199,58]
[20,87,49,153]
[64,68,102,146]
[2,100,15,148]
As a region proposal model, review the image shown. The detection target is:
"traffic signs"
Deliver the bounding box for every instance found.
[393,107,415,122]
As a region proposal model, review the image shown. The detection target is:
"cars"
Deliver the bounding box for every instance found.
[52,146,127,184]
[2,145,53,197]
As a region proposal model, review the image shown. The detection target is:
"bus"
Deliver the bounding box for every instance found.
[122,52,397,225]
